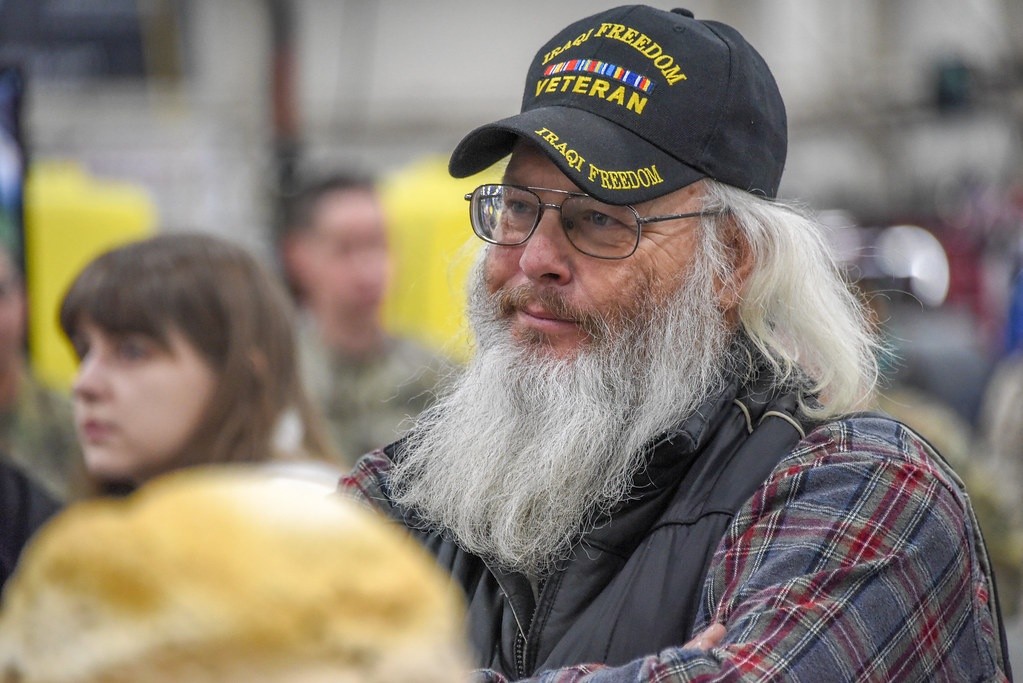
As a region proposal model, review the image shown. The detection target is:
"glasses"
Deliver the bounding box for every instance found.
[465,184,731,260]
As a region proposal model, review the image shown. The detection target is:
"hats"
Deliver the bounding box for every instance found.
[449,3,789,207]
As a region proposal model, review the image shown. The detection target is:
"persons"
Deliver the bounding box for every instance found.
[0,173,469,683]
[336,6,1013,683]
